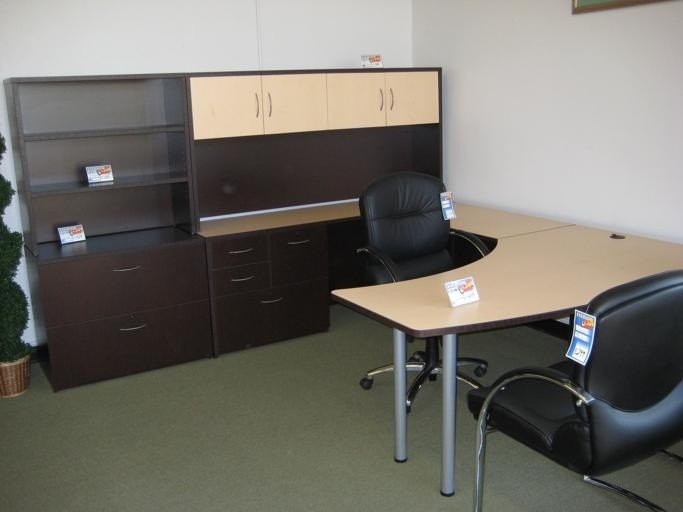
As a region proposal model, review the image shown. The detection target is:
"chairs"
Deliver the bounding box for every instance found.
[467,269,683,512]
[355,171,490,414]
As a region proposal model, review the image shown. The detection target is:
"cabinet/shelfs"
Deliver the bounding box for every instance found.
[3,73,216,394]
[184,66,444,358]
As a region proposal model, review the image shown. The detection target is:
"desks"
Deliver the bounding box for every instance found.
[331,198,682,498]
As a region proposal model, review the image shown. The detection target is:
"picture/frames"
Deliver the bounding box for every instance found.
[571,1,668,14]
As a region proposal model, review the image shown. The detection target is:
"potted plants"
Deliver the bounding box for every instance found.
[0,133,33,398]
